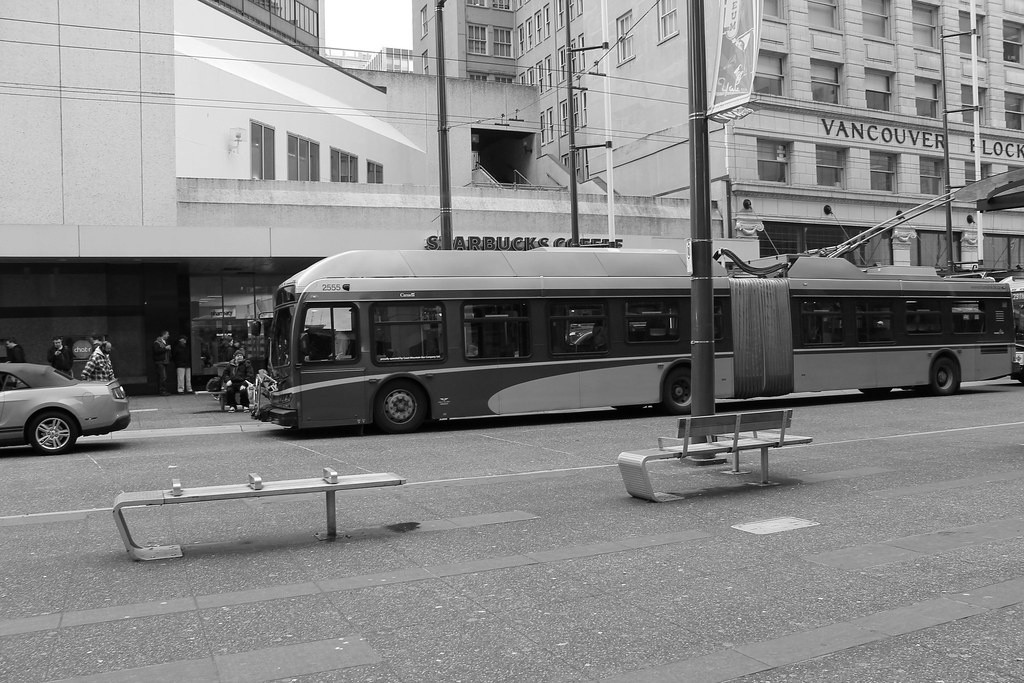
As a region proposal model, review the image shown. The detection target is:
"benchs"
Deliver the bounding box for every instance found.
[113,467,406,561]
[618,410,813,502]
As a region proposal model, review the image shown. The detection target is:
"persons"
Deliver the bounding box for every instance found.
[148,330,172,395]
[173,336,195,395]
[6,339,25,363]
[222,350,254,413]
[47,336,73,377]
[81,341,115,382]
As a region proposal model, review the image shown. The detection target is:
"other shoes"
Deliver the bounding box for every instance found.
[228,407,235,413]
[179,392,184,395]
[189,391,195,394]
[243,406,250,413]
[160,392,170,396]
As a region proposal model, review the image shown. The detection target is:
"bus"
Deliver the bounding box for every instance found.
[251,249,1017,435]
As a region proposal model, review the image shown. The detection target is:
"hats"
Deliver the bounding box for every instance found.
[234,350,243,357]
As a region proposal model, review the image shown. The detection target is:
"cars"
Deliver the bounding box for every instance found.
[0,362,132,455]
